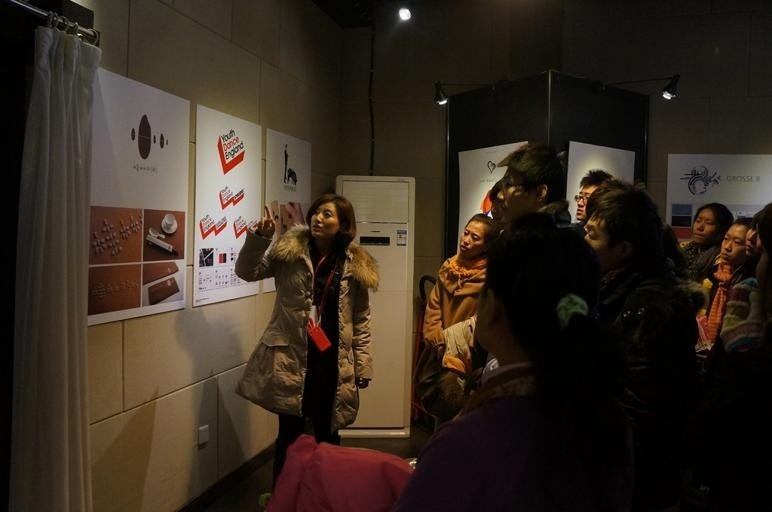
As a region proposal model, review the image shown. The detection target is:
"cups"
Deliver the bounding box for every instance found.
[164,213,176,227]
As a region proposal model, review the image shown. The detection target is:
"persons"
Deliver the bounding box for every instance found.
[230,192,378,494]
[398,210,638,511]
[489,176,503,227]
[496,137,577,233]
[421,213,494,373]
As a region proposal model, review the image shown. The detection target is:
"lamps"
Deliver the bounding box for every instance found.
[430,79,500,112]
[593,73,685,100]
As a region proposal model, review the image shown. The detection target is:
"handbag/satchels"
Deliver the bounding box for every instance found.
[411,339,468,420]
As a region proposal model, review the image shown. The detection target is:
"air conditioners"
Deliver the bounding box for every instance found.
[334,175,414,439]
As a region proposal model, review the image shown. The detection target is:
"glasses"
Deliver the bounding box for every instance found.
[574,194,591,206]
[501,178,527,192]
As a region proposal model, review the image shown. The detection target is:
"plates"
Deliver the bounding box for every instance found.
[162,218,178,234]
